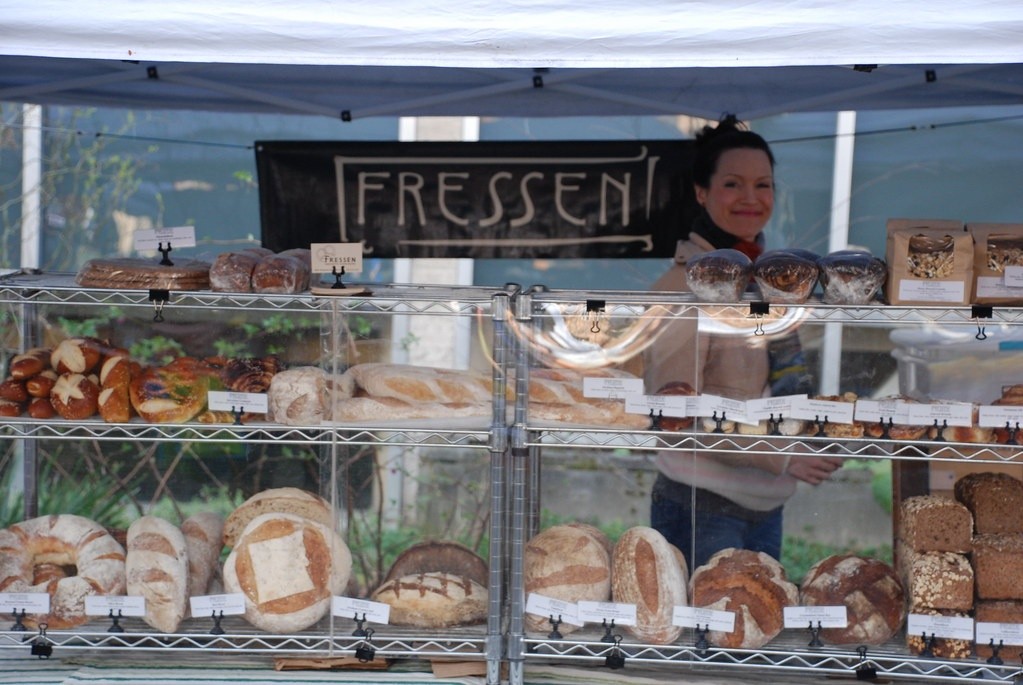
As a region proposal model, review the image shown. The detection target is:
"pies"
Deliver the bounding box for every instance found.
[75,258,211,291]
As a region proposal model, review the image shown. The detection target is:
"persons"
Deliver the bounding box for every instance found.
[643,112,842,580]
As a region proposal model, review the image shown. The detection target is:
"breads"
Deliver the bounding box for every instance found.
[366,543,489,626]
[895,471,1023,669]
[210,246,320,296]
[685,250,888,307]
[0,334,1022,447]
[521,523,907,654]
[1,486,356,634]
